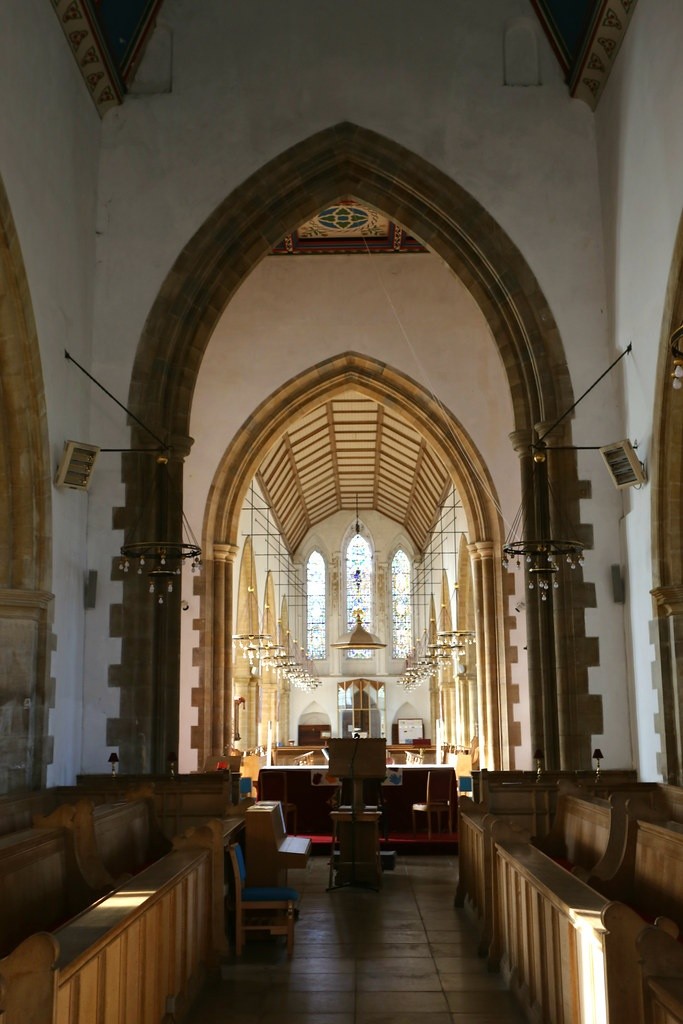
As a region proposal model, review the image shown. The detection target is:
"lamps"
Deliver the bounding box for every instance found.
[331,493,387,651]
[64,349,204,604]
[52,439,100,493]
[397,482,476,690]
[232,479,323,689]
[597,439,650,491]
[592,749,604,783]
[502,343,638,600]
[165,752,177,784]
[108,752,120,777]
[533,748,545,783]
[668,319,683,390]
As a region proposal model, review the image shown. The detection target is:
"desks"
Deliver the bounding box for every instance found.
[258,767,459,831]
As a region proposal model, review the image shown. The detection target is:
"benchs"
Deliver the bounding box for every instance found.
[0,787,295,1024]
[455,781,683,1024]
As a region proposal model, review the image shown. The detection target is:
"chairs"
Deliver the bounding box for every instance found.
[412,770,452,837]
[227,839,297,955]
[258,771,296,836]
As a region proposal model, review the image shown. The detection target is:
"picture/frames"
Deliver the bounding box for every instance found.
[397,718,424,745]
